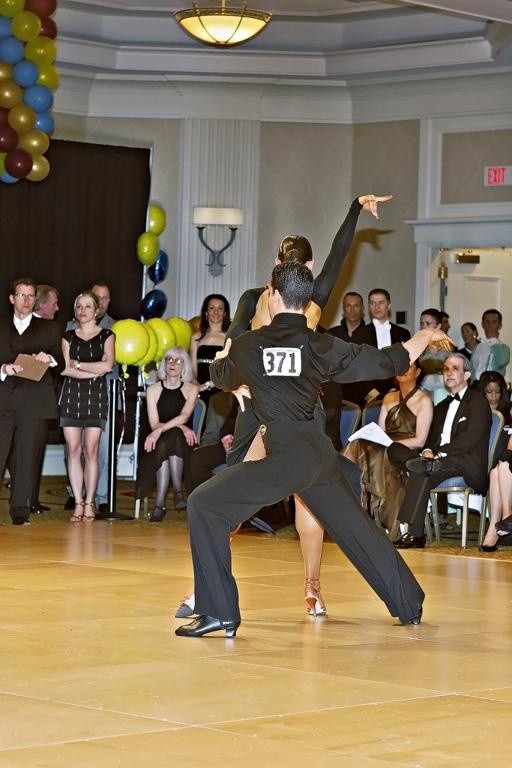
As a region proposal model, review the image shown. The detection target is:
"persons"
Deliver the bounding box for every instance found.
[226,192,395,621]
[0,281,242,527]
[315,287,512,554]
[174,259,455,640]
[316,287,512,553]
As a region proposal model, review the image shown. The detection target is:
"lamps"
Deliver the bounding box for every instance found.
[168,0,272,52]
[190,203,247,278]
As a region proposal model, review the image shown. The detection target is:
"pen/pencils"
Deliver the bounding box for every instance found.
[9,362,17,375]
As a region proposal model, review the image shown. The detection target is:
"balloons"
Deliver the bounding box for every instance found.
[137,232,159,266]
[1,0,61,186]
[148,204,166,236]
[148,250,169,284]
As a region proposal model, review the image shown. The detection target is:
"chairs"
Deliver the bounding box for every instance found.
[128,390,512,553]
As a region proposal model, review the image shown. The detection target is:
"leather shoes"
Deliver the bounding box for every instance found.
[395,533,426,548]
[174,492,186,513]
[404,456,441,475]
[149,506,167,522]
[479,534,501,551]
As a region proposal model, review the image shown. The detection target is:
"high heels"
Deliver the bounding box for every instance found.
[175,615,241,637]
[176,594,194,617]
[304,577,326,616]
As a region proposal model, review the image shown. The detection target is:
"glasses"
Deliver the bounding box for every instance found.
[166,357,183,365]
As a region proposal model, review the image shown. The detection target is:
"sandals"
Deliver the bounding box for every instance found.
[70,501,85,522]
[83,501,96,522]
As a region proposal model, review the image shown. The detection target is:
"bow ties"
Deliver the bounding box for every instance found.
[446,392,460,404]
